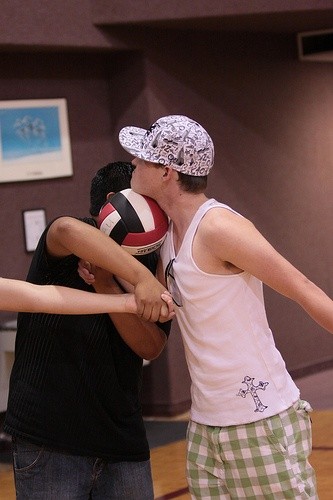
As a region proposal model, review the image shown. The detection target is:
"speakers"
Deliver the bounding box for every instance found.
[297,29,333,63]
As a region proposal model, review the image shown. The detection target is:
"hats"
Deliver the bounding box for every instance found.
[119,115,214,176]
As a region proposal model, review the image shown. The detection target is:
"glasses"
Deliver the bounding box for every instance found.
[165,258,182,307]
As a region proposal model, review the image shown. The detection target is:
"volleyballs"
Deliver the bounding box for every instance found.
[99,189,169,256]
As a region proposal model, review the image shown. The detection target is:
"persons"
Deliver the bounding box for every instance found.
[0,160,177,500]
[77,114,333,500]
